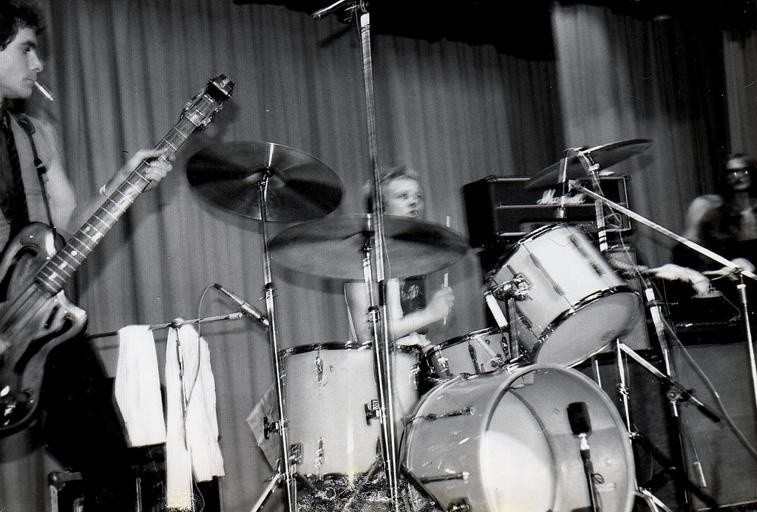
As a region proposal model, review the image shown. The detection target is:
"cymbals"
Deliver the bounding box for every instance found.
[185,140,343,223]
[267,214,467,280]
[525,138,653,189]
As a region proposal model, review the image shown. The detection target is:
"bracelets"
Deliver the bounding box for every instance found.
[97,182,108,200]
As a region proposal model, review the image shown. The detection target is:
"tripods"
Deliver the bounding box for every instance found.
[342,249,442,512]
[243,185,322,512]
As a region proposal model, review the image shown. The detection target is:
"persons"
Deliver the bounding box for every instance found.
[0,2,176,512]
[676,149,754,279]
[337,162,455,351]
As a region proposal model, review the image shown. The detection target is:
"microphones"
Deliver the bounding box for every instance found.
[312,0,346,19]
[553,155,571,223]
[567,401,593,438]
[215,282,269,328]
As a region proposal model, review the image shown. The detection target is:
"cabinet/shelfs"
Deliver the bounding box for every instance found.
[460,172,637,248]
[48,465,219,511]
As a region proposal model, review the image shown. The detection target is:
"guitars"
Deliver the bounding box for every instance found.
[1,74,235,436]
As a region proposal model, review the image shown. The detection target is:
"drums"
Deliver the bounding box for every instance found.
[279,341,421,486]
[483,222,641,368]
[400,363,637,511]
[422,327,511,379]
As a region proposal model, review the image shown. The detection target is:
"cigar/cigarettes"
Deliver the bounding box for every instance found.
[33,80,54,102]
